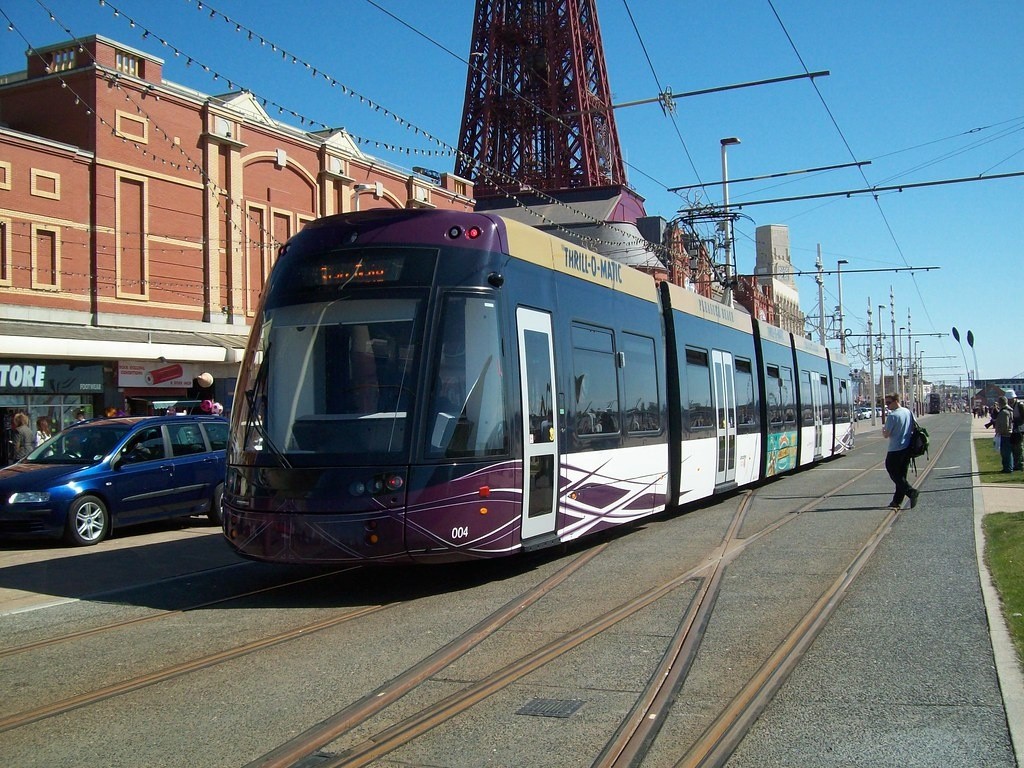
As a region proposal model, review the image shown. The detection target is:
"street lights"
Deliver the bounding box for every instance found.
[899,327,906,407]
[919,350,925,380]
[878,303,887,424]
[718,137,741,309]
[914,340,920,363]
[837,258,849,353]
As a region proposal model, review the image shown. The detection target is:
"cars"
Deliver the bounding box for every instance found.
[855,406,891,421]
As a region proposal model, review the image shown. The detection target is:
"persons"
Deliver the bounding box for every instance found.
[1004,390,1024,470]
[9,413,33,465]
[882,392,920,510]
[75,412,89,452]
[34,419,53,458]
[972,406,988,419]
[989,396,1014,473]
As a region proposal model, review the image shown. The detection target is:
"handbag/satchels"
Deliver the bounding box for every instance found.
[907,412,928,458]
[992,432,1001,453]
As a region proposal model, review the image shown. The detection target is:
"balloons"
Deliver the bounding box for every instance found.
[200,400,212,412]
[211,403,223,414]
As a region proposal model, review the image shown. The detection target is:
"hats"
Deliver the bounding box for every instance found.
[1004,390,1017,399]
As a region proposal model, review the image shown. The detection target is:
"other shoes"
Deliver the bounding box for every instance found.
[999,468,1012,473]
[1013,466,1024,471]
[910,489,919,509]
[888,502,900,510]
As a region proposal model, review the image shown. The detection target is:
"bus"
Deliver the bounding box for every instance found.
[221,206,858,569]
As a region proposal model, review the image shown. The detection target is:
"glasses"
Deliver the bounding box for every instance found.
[886,400,896,406]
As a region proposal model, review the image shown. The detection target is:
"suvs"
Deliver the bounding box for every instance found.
[0,413,230,548]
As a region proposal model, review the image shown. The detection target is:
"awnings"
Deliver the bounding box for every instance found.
[131,395,201,409]
